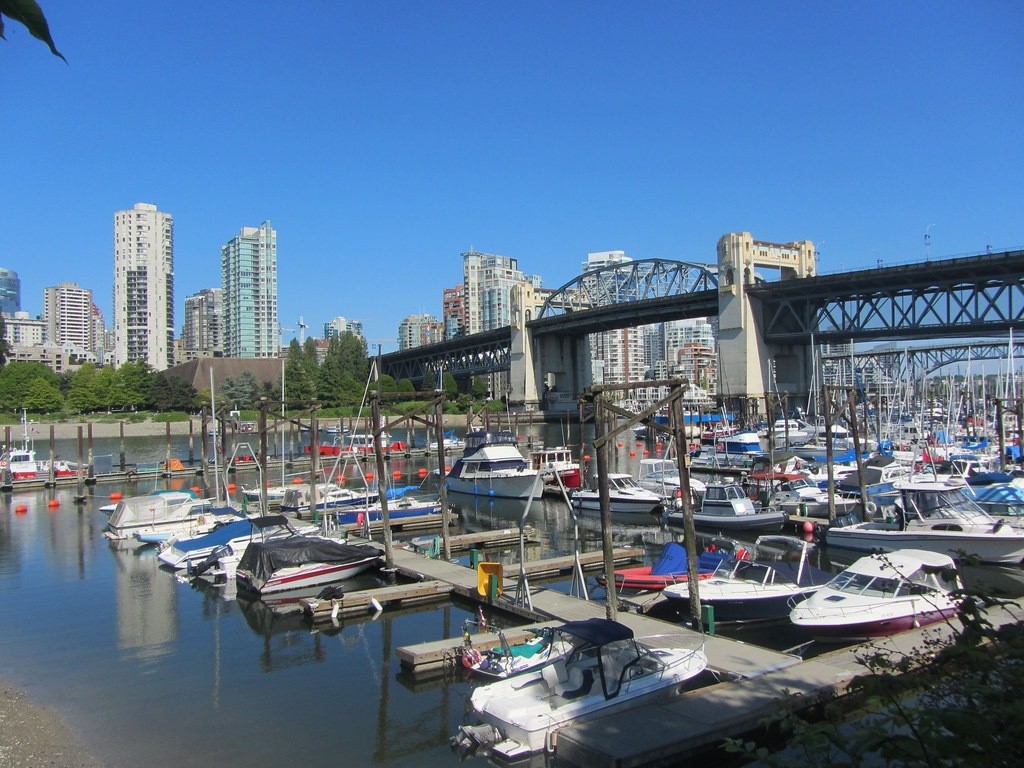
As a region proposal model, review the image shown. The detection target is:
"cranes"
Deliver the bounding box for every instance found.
[295,316,311,347]
[277,324,295,356]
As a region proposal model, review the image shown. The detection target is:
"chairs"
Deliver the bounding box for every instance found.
[568,678,612,704]
[553,669,582,696]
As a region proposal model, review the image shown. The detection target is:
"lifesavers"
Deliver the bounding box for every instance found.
[0,460,6,467]
[864,502,877,514]
[717,443,724,451]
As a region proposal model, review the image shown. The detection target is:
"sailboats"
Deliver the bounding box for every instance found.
[633,332,1023,529]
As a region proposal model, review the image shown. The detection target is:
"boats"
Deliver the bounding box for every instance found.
[570,474,662,513]
[95,490,708,767]
[598,526,985,641]
[0,406,91,486]
[242,477,458,534]
[673,480,788,537]
[527,447,590,488]
[823,478,1023,565]
[430,430,544,500]
